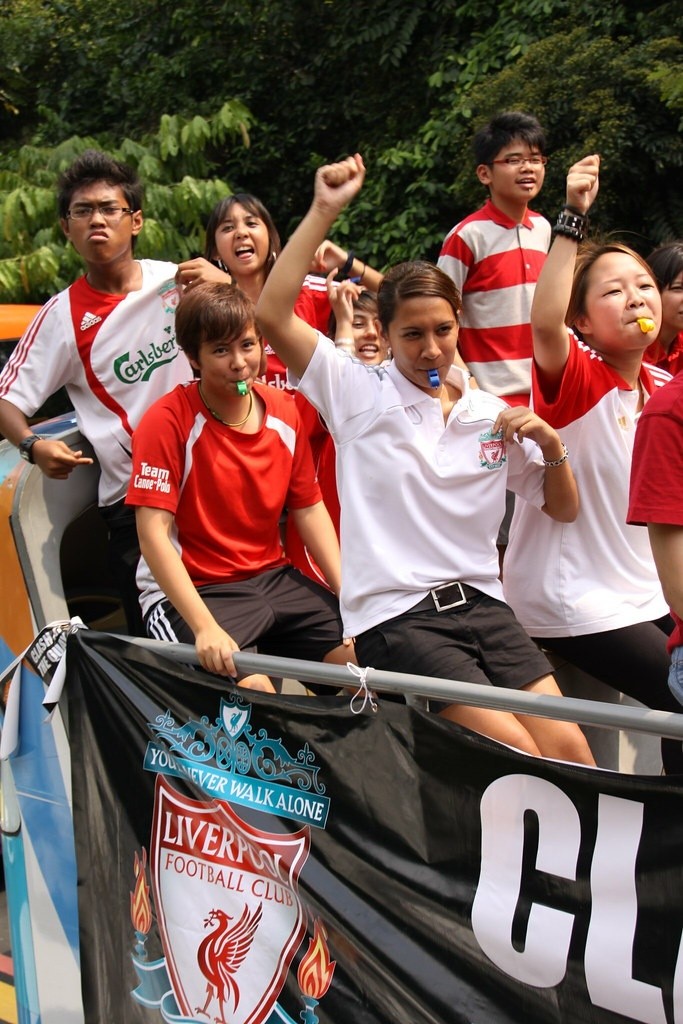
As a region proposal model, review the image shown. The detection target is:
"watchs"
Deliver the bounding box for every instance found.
[19,435,42,465]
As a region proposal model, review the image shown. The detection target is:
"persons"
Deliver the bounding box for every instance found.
[1,113,682,765]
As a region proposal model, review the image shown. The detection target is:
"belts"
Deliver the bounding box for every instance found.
[404,580,481,614]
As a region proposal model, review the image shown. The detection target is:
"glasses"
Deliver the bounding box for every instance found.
[65,207,136,221]
[486,156,547,167]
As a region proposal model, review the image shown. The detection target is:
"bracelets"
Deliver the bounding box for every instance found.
[335,338,354,345]
[231,276,237,286]
[336,346,356,352]
[361,262,365,277]
[561,202,586,219]
[557,212,585,232]
[542,442,568,466]
[553,225,584,243]
[340,251,355,274]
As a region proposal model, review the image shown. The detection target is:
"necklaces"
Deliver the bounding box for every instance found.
[199,382,253,427]
